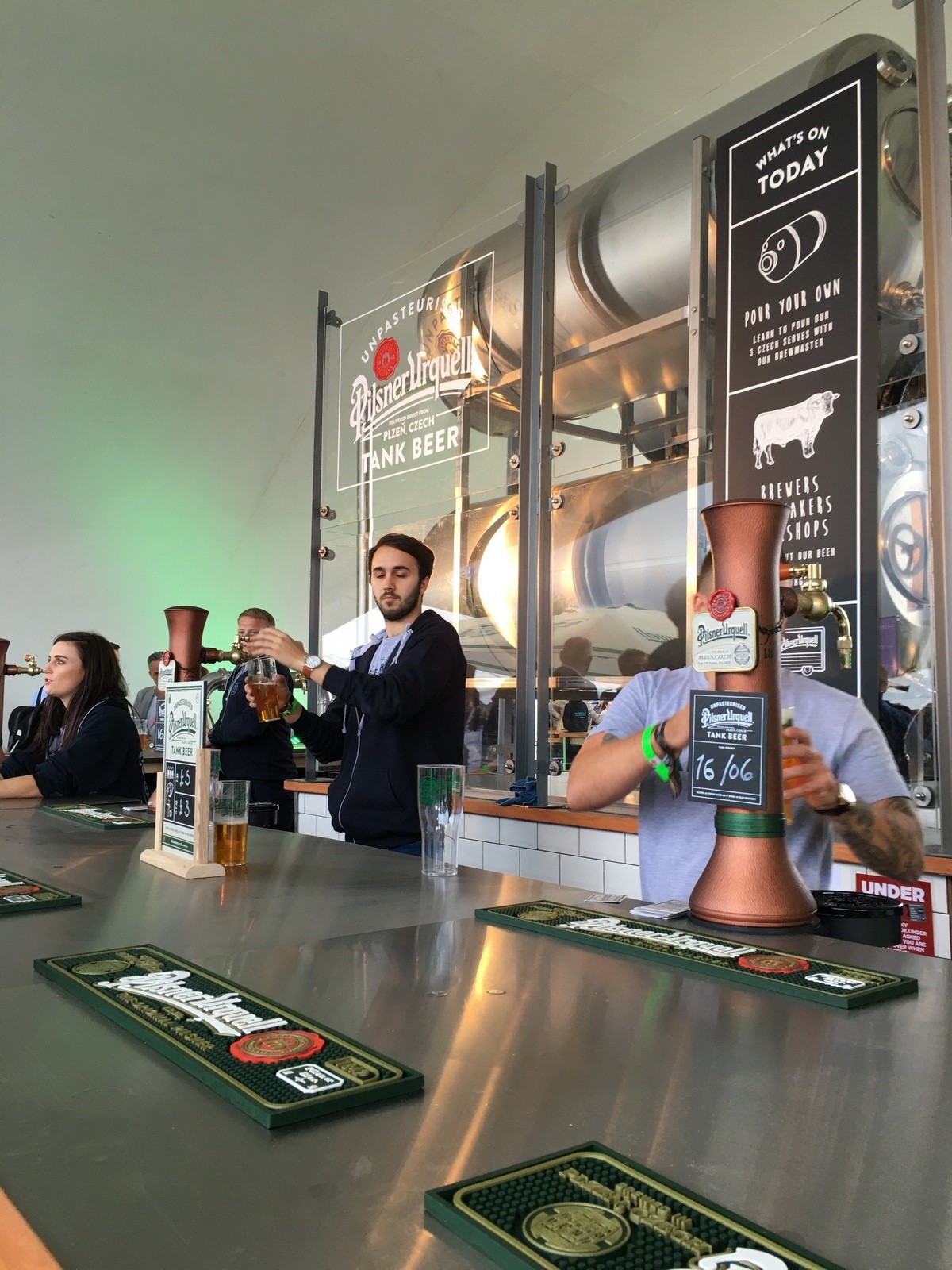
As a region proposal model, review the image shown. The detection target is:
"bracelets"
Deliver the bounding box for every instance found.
[642,722,683,797]
[279,695,299,717]
[481,765,489,772]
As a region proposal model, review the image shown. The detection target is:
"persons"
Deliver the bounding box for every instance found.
[205,608,298,832]
[0,631,151,805]
[464,637,651,775]
[878,666,918,783]
[564,551,925,905]
[244,533,467,861]
[131,651,165,746]
[645,577,686,670]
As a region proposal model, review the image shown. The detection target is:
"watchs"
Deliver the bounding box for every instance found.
[302,654,321,679]
[814,782,856,815]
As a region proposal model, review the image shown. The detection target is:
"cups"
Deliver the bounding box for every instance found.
[210,750,220,828]
[417,764,465,877]
[245,656,280,723]
[131,716,149,750]
[213,779,249,867]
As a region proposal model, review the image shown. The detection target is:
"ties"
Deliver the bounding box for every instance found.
[219,667,242,725]
